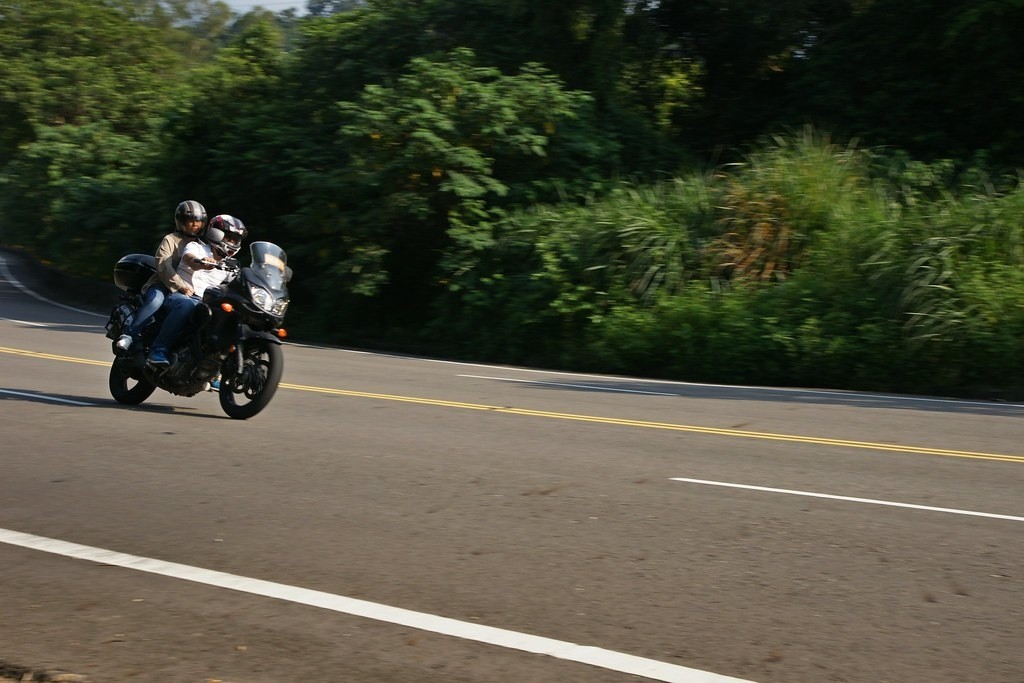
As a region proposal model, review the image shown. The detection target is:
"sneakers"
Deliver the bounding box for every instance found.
[146,348,171,366]
[211,379,220,391]
[116,334,132,350]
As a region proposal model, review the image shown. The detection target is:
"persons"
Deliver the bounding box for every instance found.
[116,199,206,350]
[146,213,248,369]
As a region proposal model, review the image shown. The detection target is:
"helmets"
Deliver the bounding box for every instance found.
[175,200,207,232]
[203,214,248,256]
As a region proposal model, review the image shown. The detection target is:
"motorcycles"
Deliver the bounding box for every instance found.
[105,240,293,420]
